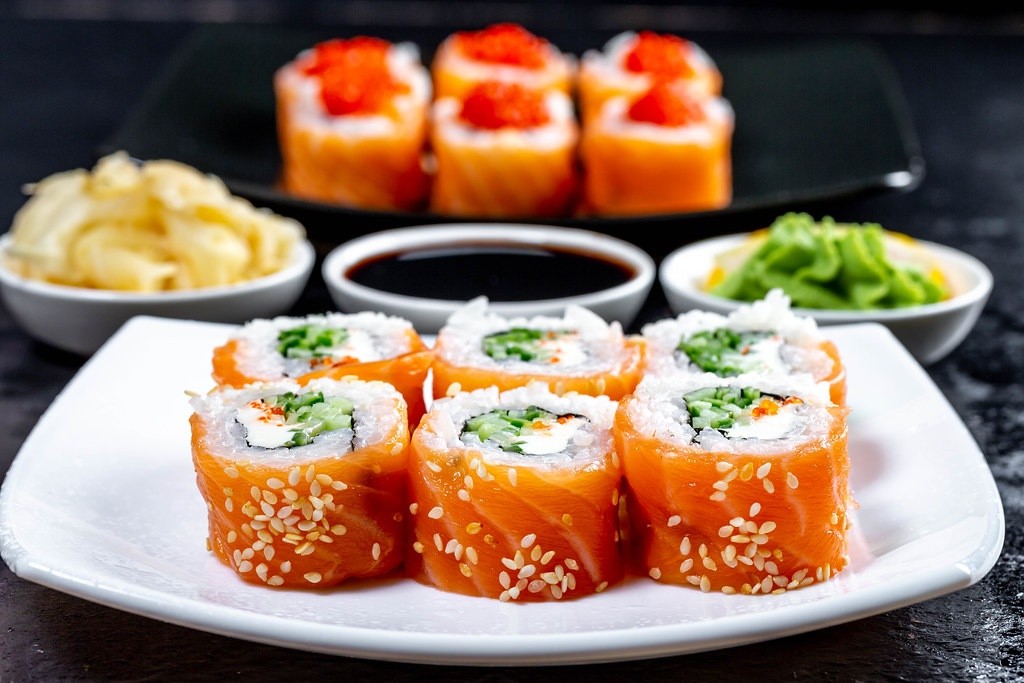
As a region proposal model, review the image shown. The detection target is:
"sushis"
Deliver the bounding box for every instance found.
[184,21,852,606]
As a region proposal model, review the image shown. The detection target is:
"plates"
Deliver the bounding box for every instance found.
[222,15,920,250]
[0,315,1002,667]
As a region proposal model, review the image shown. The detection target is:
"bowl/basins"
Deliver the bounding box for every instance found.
[0,225,314,358]
[660,219,993,378]
[327,216,657,337]
